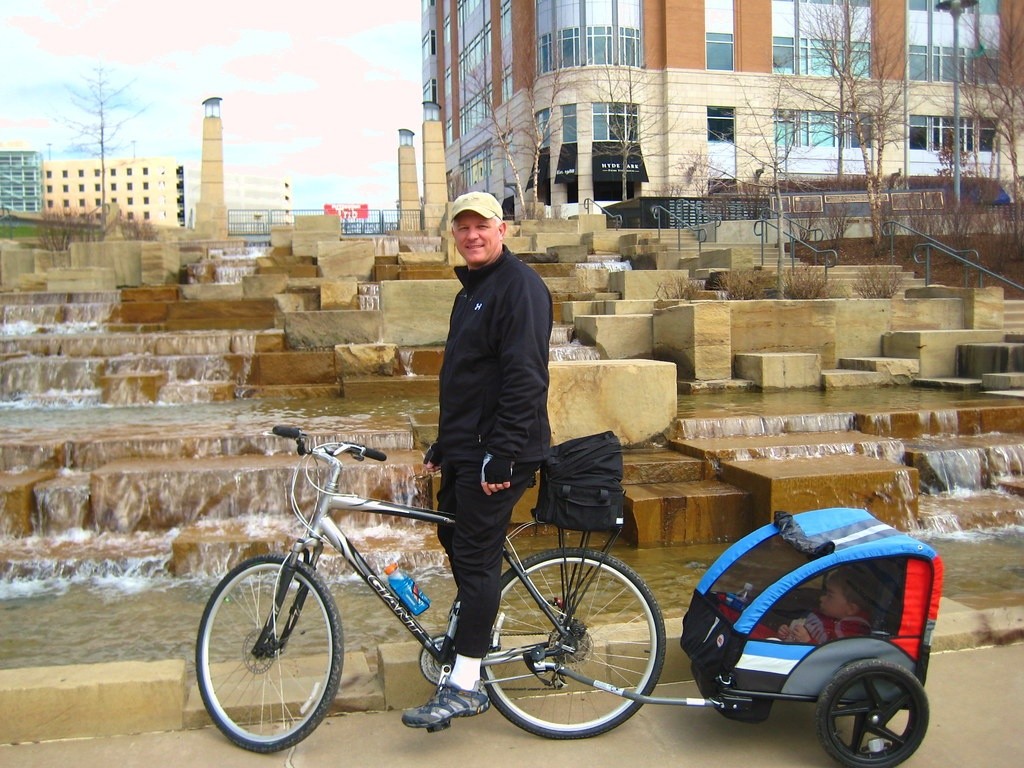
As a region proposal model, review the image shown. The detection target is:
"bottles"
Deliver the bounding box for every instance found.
[733,582,756,611]
[384,562,430,617]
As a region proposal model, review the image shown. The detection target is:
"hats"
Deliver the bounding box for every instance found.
[449,191,504,223]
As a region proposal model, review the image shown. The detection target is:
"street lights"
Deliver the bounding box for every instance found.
[937,0,978,209]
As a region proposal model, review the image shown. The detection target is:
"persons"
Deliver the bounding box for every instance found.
[401,193,553,727]
[718,567,878,645]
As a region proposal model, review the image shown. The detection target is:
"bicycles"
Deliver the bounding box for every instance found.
[193,422,669,755]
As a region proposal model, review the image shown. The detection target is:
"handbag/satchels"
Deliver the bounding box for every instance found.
[536,430,628,532]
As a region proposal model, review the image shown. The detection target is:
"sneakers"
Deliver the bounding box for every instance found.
[400,676,491,729]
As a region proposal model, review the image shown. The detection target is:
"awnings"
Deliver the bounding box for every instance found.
[525,146,550,192]
[554,143,578,183]
[592,142,649,182]
